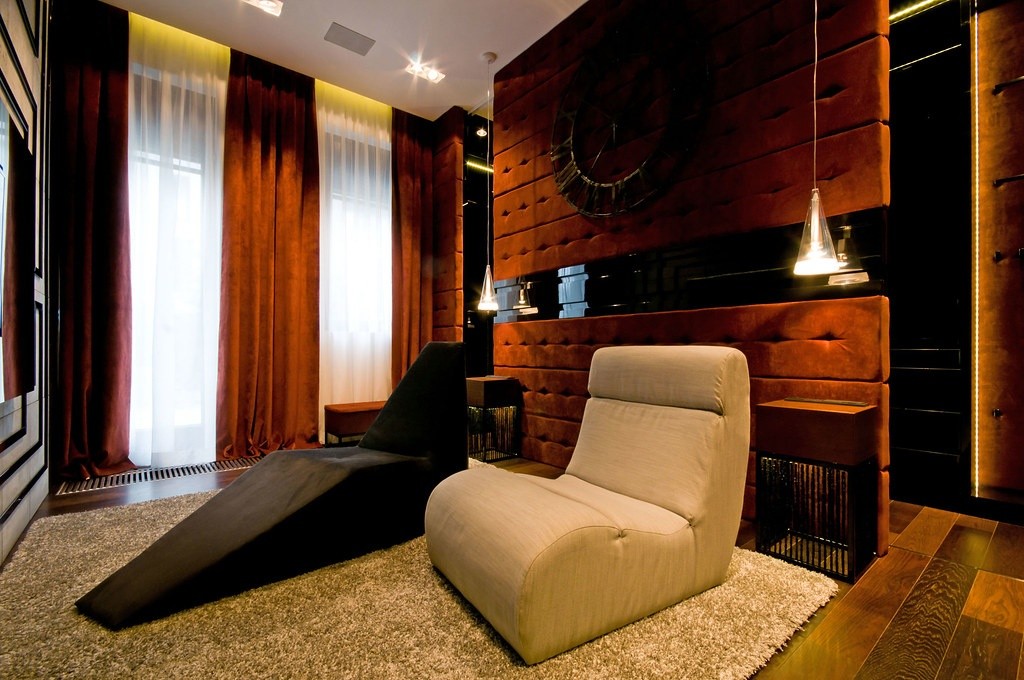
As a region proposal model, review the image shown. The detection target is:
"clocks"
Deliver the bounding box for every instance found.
[552,6,715,220]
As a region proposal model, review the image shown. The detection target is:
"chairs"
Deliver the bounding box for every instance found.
[427,342,750,662]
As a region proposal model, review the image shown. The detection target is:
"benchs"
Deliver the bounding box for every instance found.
[324,401,386,451]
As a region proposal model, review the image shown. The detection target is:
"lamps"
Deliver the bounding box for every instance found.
[826,215,868,286]
[793,2,839,276]
[477,49,501,311]
[509,280,538,315]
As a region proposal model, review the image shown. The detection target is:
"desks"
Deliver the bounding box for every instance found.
[756,398,876,582]
[466,377,520,464]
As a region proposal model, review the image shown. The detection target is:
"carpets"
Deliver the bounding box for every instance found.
[0,449,838,680]
